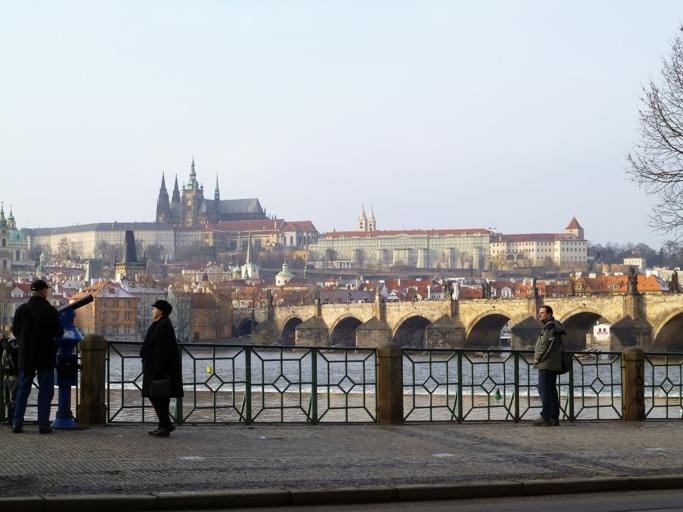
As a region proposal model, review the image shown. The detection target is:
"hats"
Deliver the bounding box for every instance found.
[150,300,172,314]
[31,280,52,291]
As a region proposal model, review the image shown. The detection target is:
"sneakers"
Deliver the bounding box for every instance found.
[533,417,559,426]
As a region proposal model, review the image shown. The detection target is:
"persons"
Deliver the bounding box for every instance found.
[11,280,64,433]
[532,305,562,426]
[140,300,184,437]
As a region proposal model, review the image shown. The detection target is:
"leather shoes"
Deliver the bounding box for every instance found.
[11,426,23,433]
[39,425,53,434]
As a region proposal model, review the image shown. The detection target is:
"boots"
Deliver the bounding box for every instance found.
[148,422,175,436]
